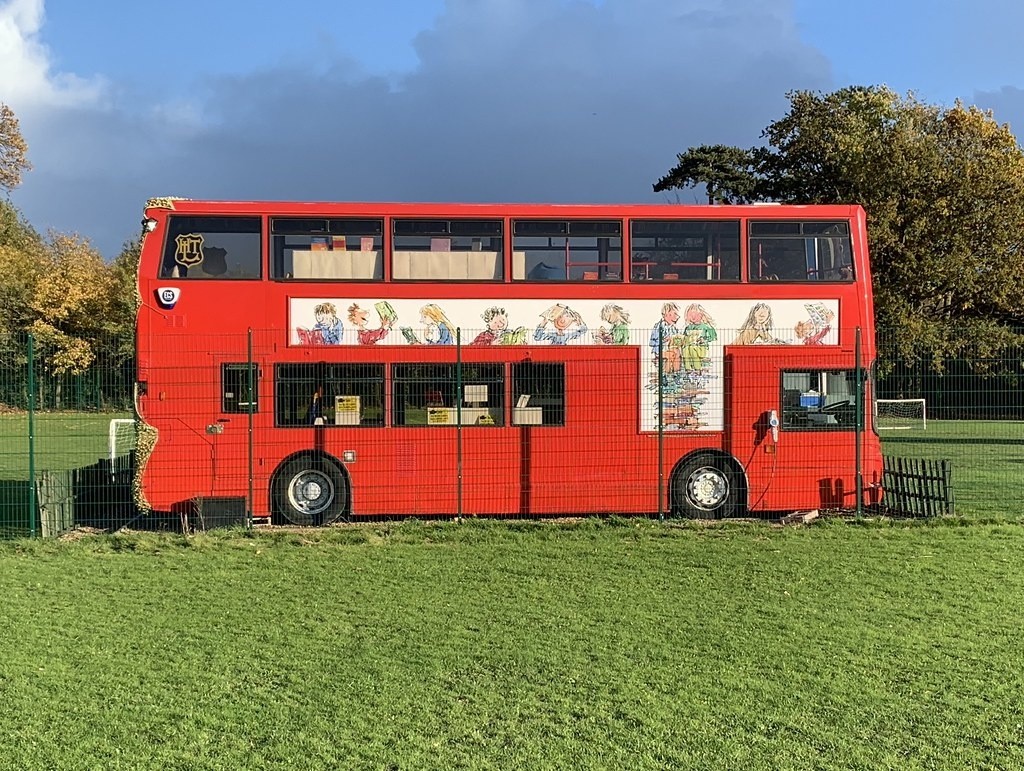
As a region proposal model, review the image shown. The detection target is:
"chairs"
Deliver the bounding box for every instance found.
[304,387,323,424]
[751,274,778,281]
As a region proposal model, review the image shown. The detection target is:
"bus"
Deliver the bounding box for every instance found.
[134,196,880,533]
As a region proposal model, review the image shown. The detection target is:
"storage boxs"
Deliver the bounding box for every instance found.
[800,396,826,406]
